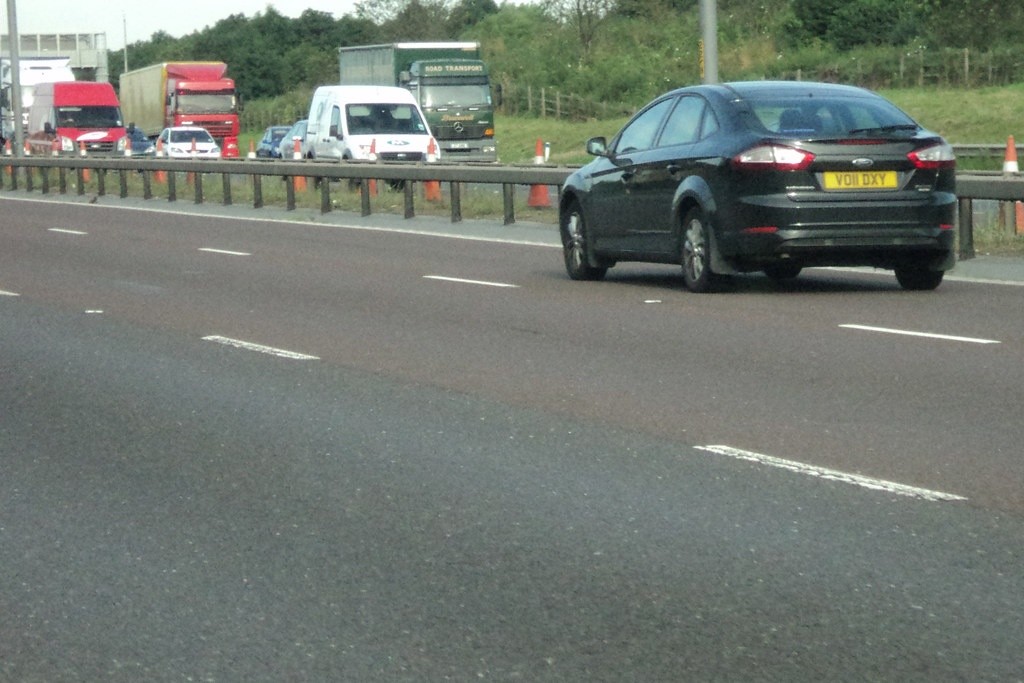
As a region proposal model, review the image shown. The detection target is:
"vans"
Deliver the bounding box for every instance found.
[26,80,135,153]
[304,85,441,192]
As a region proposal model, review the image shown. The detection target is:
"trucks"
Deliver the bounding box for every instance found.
[119,61,247,160]
[337,39,504,165]
[0,57,83,136]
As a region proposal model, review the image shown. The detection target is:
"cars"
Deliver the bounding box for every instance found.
[256,126,292,158]
[277,118,309,181]
[555,79,962,296]
[154,126,222,162]
[126,125,154,154]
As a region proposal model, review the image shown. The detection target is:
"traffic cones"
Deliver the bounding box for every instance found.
[290,139,307,194]
[247,139,258,159]
[5,139,13,175]
[355,138,378,198]
[422,138,442,201]
[995,133,1024,235]
[153,138,167,184]
[186,137,199,188]
[525,137,552,209]
[124,137,132,157]
[77,140,91,184]
[24,141,30,155]
[51,141,59,170]
